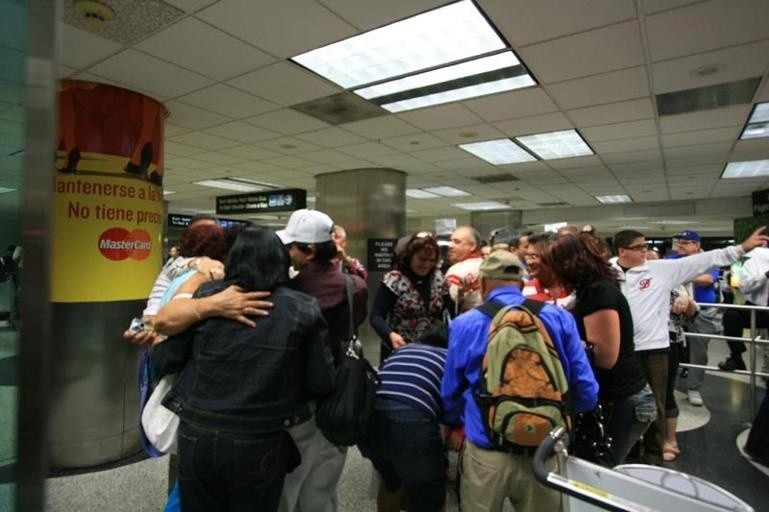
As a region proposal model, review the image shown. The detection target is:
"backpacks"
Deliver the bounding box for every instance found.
[474,299,575,447]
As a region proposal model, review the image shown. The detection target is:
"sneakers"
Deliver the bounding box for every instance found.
[718,358,746,370]
[687,388,704,406]
[663,449,679,461]
[680,369,688,378]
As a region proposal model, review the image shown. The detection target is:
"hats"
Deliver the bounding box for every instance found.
[480,250,523,281]
[274,209,334,245]
[674,230,701,240]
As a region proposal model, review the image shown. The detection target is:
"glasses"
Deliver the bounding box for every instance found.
[675,241,690,245]
[624,244,648,250]
[406,231,432,245]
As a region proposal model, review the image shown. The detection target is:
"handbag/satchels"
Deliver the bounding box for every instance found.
[576,402,615,469]
[142,374,180,455]
[316,336,381,446]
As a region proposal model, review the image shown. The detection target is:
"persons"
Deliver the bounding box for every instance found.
[124,208,767,510]
